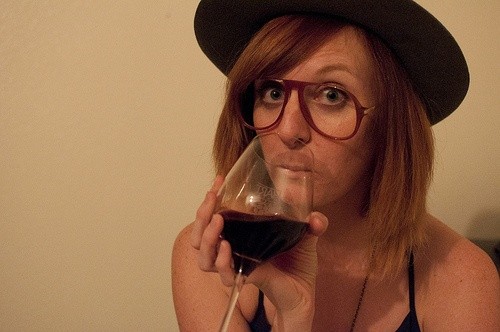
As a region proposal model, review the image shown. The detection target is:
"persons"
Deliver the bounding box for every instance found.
[170,0,500,332]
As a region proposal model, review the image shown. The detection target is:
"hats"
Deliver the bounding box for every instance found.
[193,0,470,127]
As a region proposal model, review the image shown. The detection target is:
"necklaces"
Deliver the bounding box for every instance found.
[344,240,378,332]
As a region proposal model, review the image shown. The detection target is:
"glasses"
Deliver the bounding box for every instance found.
[236,77,386,140]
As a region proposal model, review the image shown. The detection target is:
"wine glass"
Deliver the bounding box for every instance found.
[208,133,315,331]
